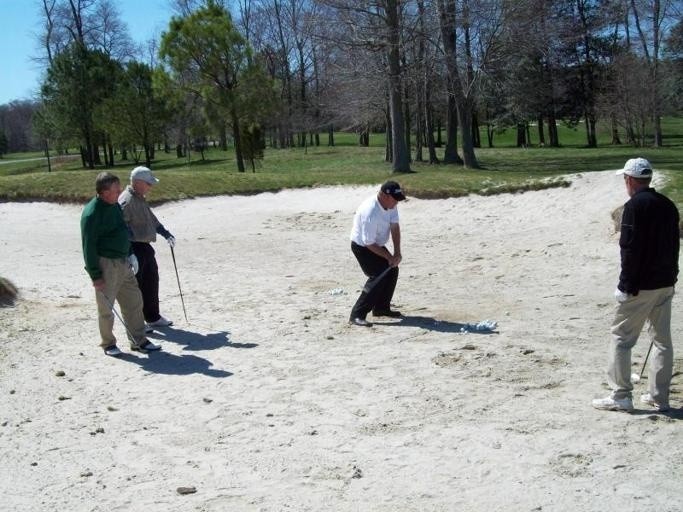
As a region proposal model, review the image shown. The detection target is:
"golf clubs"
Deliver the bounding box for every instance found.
[168,242,195,325]
[632,341,653,379]
[361,263,394,292]
[102,291,148,352]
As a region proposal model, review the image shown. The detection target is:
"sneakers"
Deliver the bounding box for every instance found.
[640,393,670,412]
[373,308,401,317]
[349,317,372,327]
[147,317,173,327]
[145,325,153,334]
[130,340,161,351]
[592,396,634,413]
[104,344,123,357]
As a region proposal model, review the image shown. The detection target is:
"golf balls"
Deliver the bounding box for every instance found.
[505,311,509,315]
[460,320,497,334]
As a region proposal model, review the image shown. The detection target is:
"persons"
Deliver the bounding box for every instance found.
[115,165,175,334]
[80,172,162,357]
[589,156,680,413]
[345,180,405,326]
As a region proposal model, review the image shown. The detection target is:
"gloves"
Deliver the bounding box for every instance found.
[167,236,175,247]
[128,254,139,276]
[614,288,634,302]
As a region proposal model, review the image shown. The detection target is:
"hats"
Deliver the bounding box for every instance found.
[381,180,406,201]
[129,166,159,184]
[615,157,653,178]
